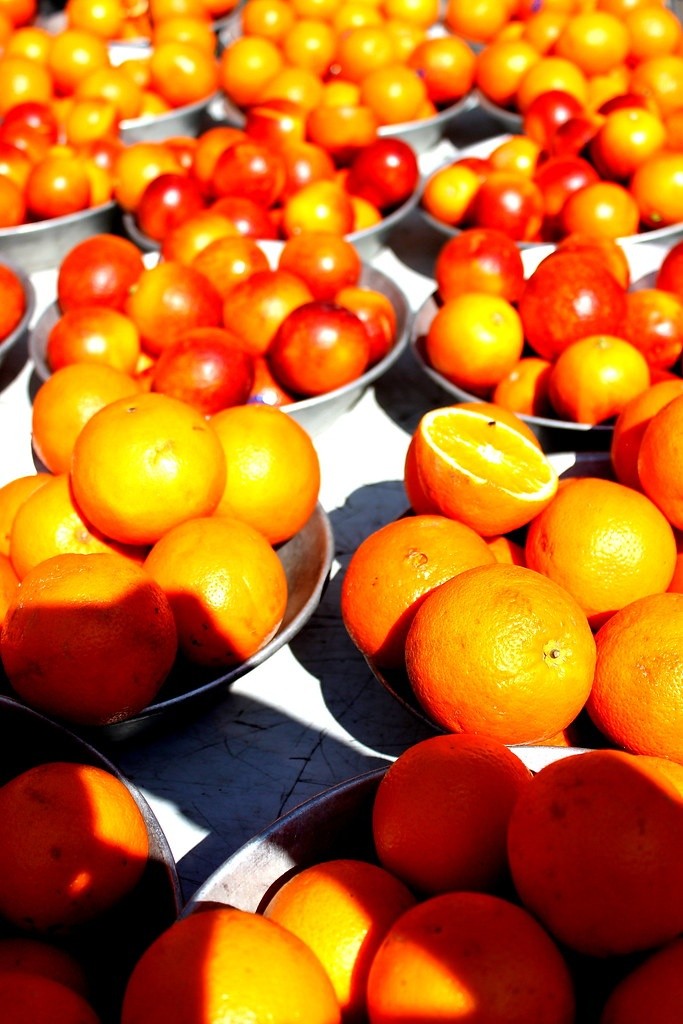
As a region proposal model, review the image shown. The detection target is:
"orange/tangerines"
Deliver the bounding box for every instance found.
[0,362,683,1024]
[0,0,683,137]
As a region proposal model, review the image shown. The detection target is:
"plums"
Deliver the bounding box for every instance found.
[0,87,683,426]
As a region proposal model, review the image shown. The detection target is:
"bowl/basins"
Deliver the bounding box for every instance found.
[364,462,621,747]
[18,499,335,748]
[181,748,599,916]
[1,7,682,433]
[0,695,182,1022]
